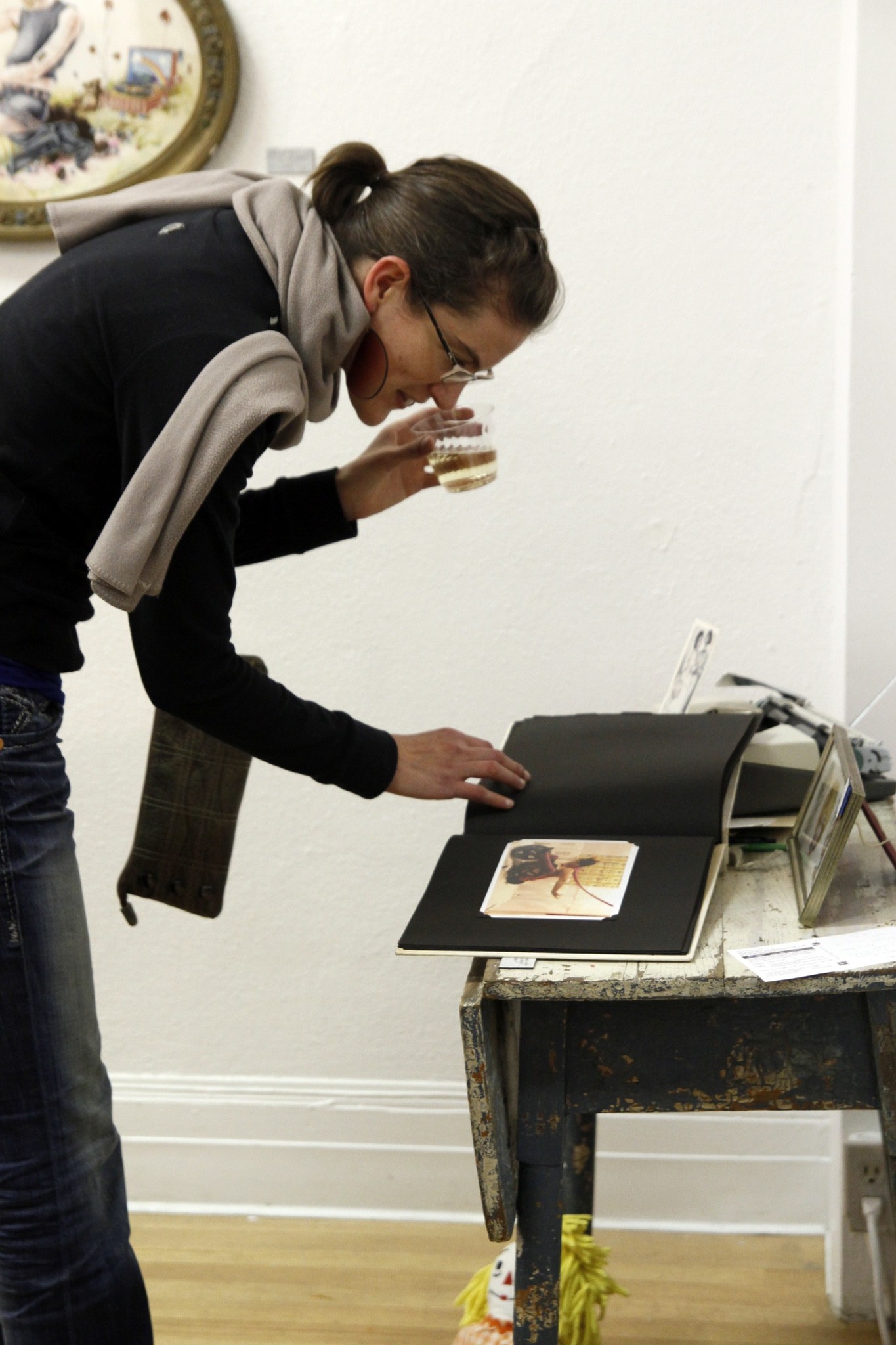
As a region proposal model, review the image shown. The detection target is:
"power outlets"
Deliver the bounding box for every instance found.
[843,1129,893,1234]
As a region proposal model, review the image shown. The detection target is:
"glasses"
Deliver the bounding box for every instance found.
[421,298,494,385]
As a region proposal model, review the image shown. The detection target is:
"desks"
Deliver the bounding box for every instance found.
[461,807,896,1345]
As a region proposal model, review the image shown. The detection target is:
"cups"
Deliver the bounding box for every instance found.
[409,401,500,494]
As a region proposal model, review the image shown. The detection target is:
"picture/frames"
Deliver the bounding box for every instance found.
[787,723,866,927]
[1,0,241,241]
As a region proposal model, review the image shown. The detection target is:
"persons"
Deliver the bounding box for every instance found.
[0,142,557,1345]
[517,857,596,898]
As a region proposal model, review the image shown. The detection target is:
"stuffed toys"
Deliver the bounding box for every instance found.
[453,1213,624,1345]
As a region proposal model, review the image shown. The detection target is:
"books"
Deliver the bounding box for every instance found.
[395,712,760,961]
